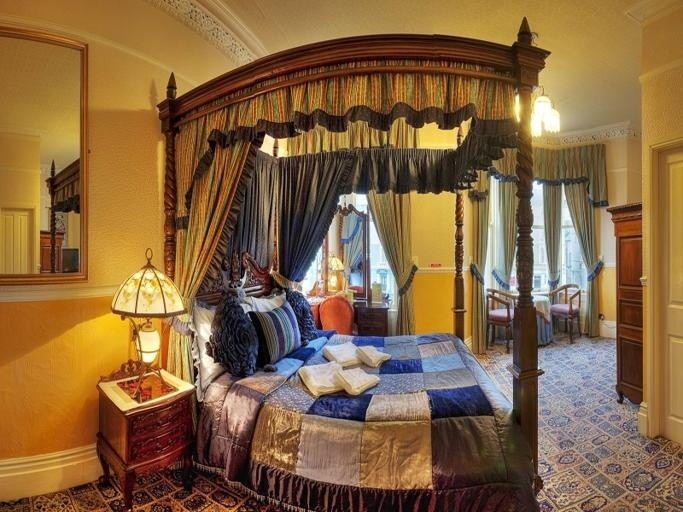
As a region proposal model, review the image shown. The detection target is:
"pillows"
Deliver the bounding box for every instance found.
[189,289,320,402]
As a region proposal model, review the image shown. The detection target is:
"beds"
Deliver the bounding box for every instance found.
[45,158,80,273]
[156,15,553,512]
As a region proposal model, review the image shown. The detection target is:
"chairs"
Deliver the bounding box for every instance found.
[486,284,581,354]
[319,297,354,335]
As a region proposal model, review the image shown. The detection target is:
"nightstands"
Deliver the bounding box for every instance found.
[95,360,196,509]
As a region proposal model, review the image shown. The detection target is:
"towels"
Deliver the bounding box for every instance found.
[299,342,392,396]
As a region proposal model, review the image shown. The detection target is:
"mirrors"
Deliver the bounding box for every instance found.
[322,204,370,303]
[0,27,90,287]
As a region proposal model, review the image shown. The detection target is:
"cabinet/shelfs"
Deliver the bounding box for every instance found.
[354,303,390,337]
[607,201,642,405]
[40,231,64,273]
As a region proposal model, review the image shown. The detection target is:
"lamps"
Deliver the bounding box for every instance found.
[111,247,187,398]
[328,254,345,287]
[515,84,561,138]
